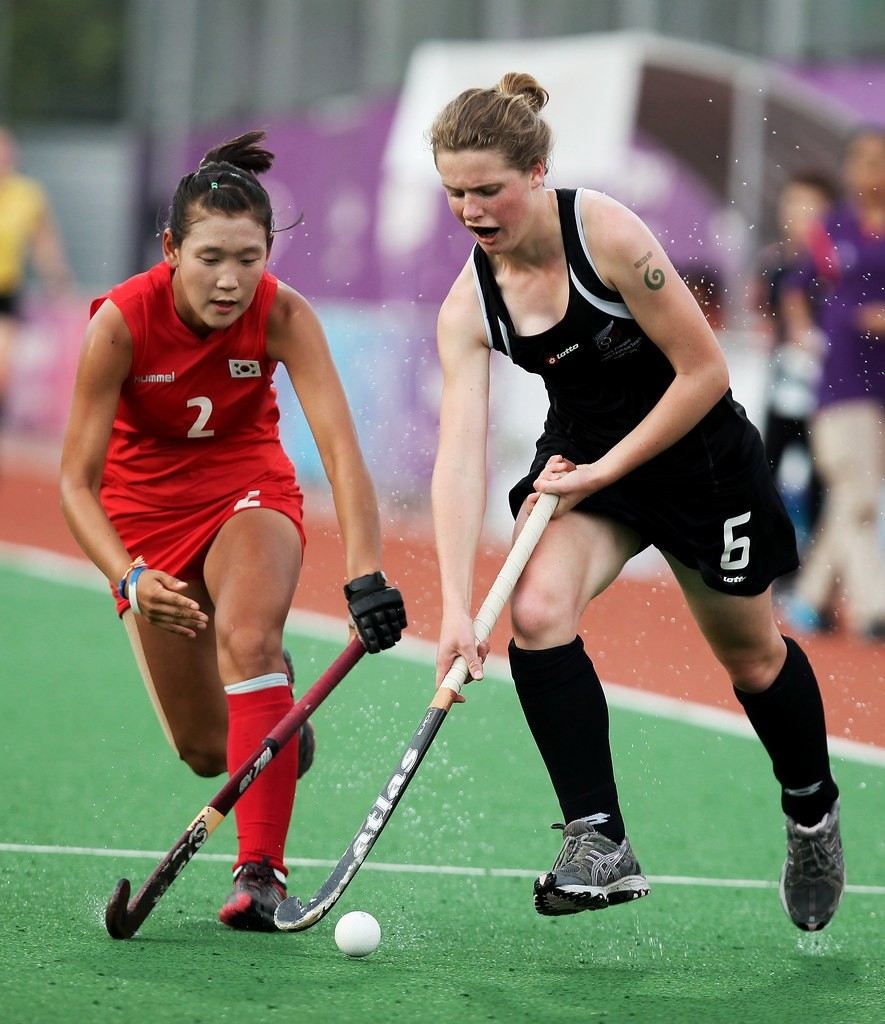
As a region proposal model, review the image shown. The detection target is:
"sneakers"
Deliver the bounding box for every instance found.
[780,795,844,930]
[531,822,651,916]
[218,861,289,931]
[281,646,315,781]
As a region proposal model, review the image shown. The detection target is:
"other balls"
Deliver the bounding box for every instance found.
[334,911,381,957]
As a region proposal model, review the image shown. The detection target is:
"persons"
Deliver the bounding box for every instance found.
[430,70,845,934]
[0,125,67,437]
[748,118,885,639]
[57,128,411,934]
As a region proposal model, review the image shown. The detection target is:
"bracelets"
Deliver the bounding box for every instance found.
[117,555,148,616]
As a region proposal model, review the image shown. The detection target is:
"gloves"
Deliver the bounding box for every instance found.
[344,569,409,655]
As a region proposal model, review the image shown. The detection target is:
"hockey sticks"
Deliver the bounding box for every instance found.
[104,635,367,940]
[271,472,571,932]
